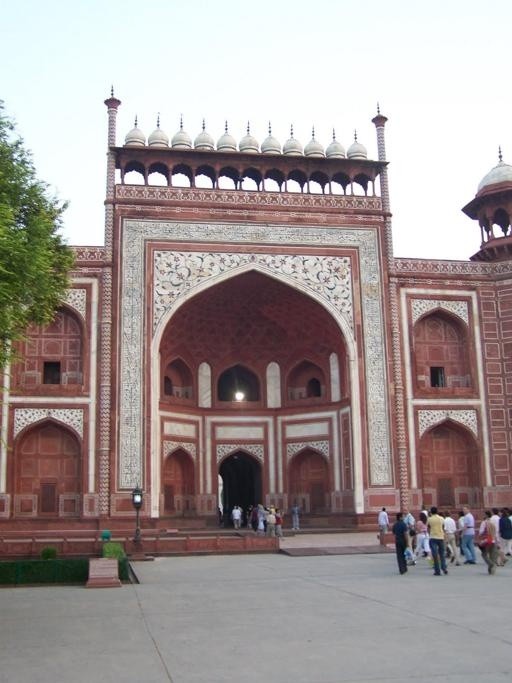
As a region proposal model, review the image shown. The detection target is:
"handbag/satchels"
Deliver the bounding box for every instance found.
[478,535,492,548]
[409,530,415,536]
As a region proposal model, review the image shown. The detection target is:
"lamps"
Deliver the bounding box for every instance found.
[130,483,145,541]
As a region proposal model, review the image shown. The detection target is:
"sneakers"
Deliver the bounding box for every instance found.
[434,569,447,575]
[488,559,508,574]
[451,553,476,566]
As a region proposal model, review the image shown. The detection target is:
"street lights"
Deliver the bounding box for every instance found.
[131,483,143,542]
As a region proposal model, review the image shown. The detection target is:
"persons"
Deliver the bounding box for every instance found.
[229,503,303,541]
[378,503,512,574]
